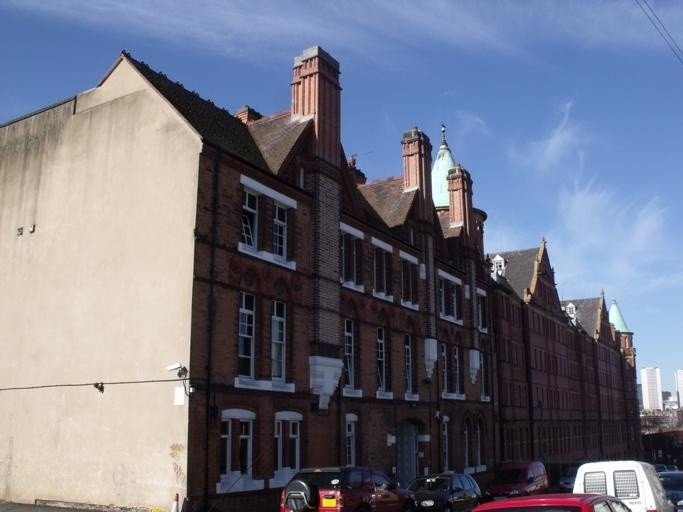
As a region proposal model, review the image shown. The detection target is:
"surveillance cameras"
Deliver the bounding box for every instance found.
[167,362,182,371]
